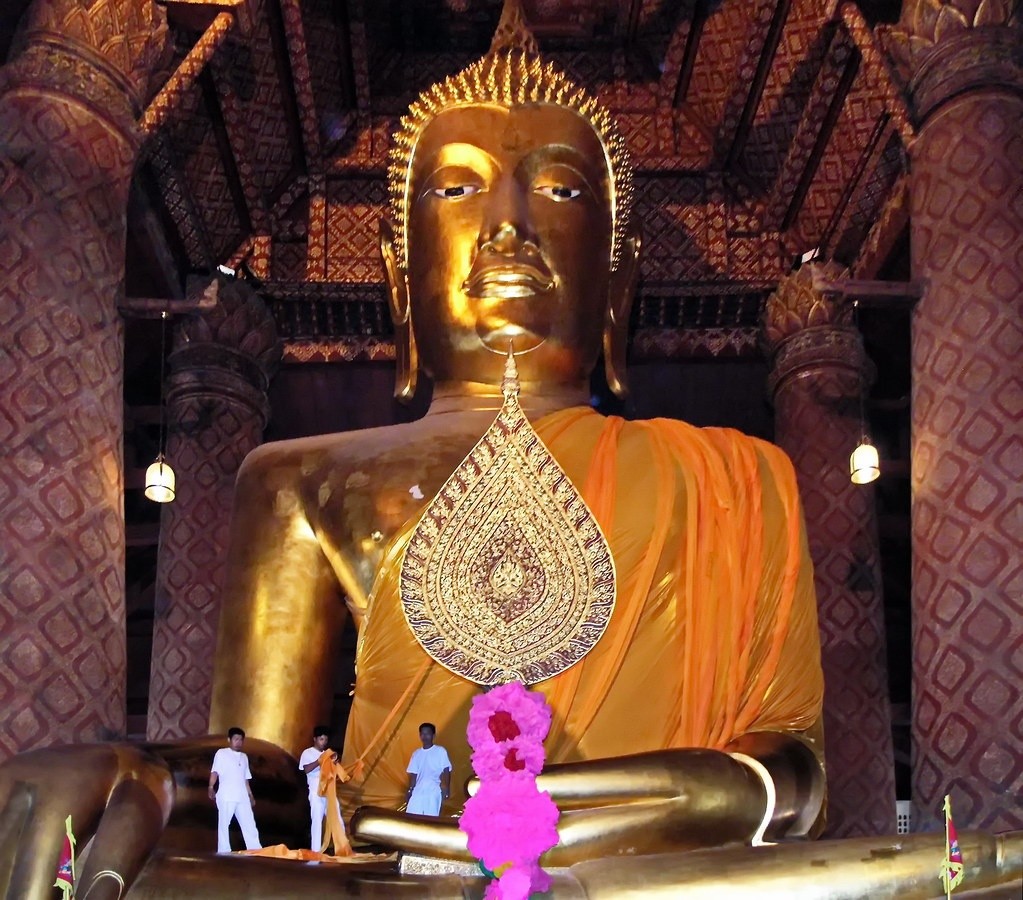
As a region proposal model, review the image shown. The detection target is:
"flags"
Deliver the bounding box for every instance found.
[52,822,77,900]
[938,795,965,894]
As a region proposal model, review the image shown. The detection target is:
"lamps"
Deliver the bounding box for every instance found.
[849,295,880,484]
[145,310,175,502]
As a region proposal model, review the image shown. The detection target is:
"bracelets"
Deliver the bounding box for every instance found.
[209,786,214,788]
[445,794,449,798]
[407,786,413,793]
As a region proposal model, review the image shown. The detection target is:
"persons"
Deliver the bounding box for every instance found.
[404,722,453,815]
[208,727,263,853]
[298,726,346,853]
[0,1,1022,899]
